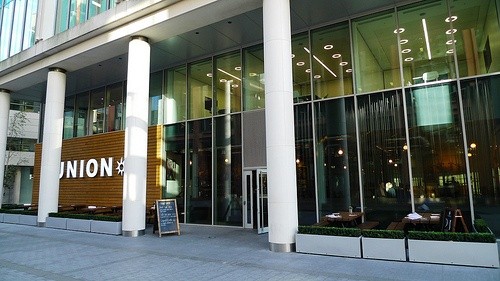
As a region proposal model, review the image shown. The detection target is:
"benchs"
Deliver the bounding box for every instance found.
[386,222,407,230]
[358,222,380,229]
[312,222,331,227]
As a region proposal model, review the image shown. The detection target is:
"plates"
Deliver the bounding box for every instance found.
[332,212,340,214]
[430,216,440,218]
[349,214,357,216]
[431,214,440,215]
[326,215,342,218]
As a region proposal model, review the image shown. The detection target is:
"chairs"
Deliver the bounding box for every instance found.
[472,219,494,235]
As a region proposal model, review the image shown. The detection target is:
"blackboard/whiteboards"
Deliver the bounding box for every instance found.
[155,199,179,233]
[443,207,468,233]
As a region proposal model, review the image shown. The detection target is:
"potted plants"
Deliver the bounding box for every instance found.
[0,208,37,226]
[407,230,500,268]
[295,225,362,259]
[361,228,406,261]
[45,212,123,235]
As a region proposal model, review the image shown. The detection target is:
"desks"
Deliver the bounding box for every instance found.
[403,212,441,231]
[58,203,121,217]
[323,211,366,228]
[15,204,37,210]
